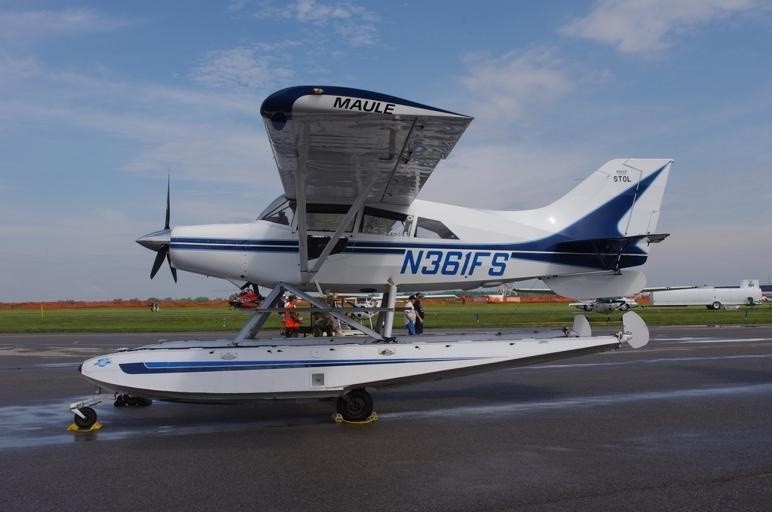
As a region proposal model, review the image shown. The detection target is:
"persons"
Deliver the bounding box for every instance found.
[156,302,160,313]
[282,294,303,331]
[313,294,335,336]
[404,296,423,335]
[413,293,424,333]
[150,299,155,312]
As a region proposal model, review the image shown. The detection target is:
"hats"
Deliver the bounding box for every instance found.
[408,293,424,299]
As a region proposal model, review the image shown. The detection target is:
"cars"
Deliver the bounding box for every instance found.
[576,295,639,313]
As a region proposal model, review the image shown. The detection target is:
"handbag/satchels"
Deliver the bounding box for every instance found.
[315,316,333,331]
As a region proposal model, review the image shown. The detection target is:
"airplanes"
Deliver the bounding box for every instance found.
[226,288,263,311]
[315,293,461,329]
[66,83,676,433]
